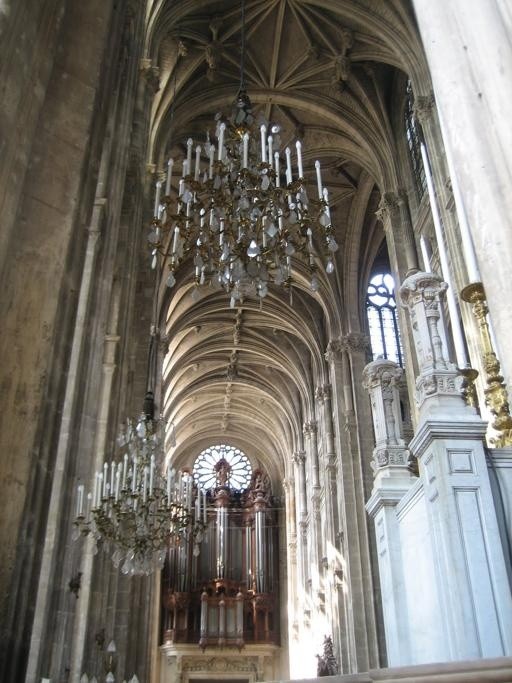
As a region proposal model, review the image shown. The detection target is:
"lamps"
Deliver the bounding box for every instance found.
[71,323,206,578]
[145,0,341,309]
